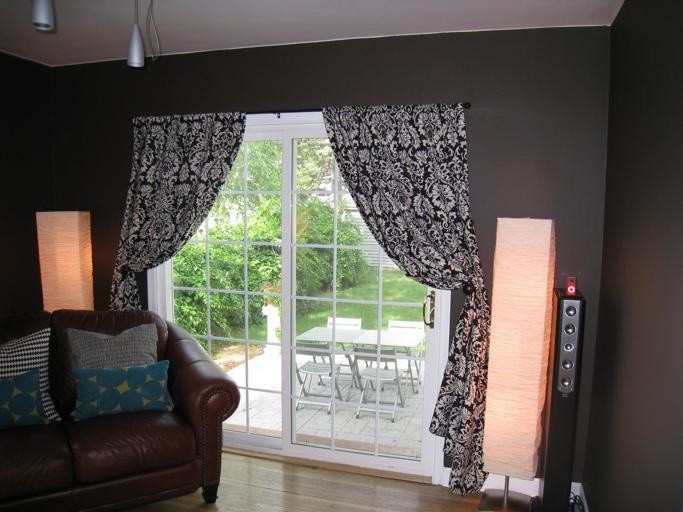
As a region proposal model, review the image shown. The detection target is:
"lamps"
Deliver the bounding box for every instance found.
[35,211,94,314]
[125,0,145,69]
[477,217,557,512]
[29,1,57,33]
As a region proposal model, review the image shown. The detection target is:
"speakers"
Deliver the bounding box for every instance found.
[537,286,588,511]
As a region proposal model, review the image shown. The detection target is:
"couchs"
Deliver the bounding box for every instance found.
[0,307,240,511]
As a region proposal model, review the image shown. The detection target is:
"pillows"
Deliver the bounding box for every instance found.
[0,321,174,428]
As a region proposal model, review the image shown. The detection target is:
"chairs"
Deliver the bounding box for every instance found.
[290,338,343,416]
[325,315,361,403]
[351,347,404,424]
[386,320,424,395]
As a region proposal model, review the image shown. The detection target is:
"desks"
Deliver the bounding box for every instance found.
[296,326,423,404]
